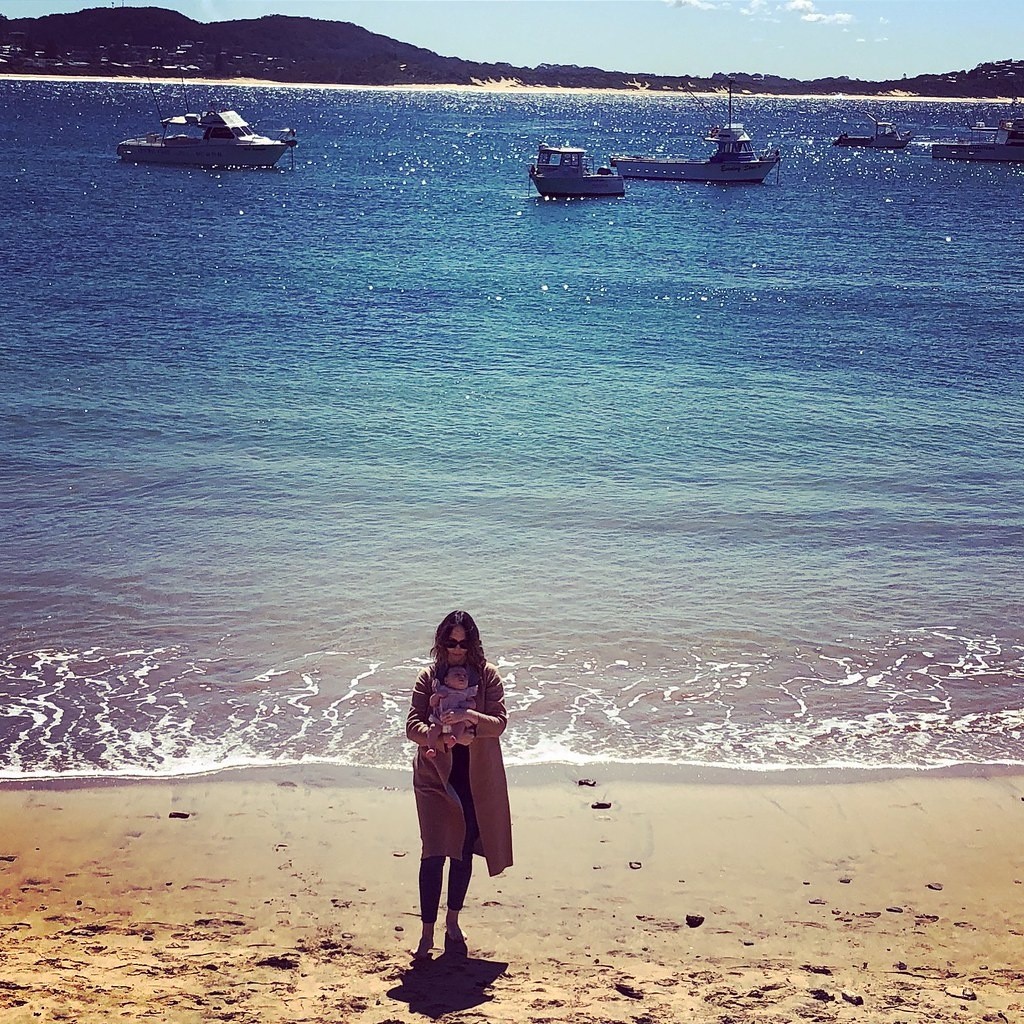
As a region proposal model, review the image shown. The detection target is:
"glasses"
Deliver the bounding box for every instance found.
[446,637,467,649]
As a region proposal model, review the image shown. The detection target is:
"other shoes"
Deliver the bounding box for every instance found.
[579,779,596,786]
[592,802,611,808]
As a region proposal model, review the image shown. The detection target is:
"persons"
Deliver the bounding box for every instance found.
[425,665,478,758]
[406,608,515,958]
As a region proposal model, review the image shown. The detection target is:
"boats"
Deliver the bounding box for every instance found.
[608,123,781,185]
[529,145,626,197]
[115,110,291,168]
[932,117,1024,162]
[834,121,910,149]
